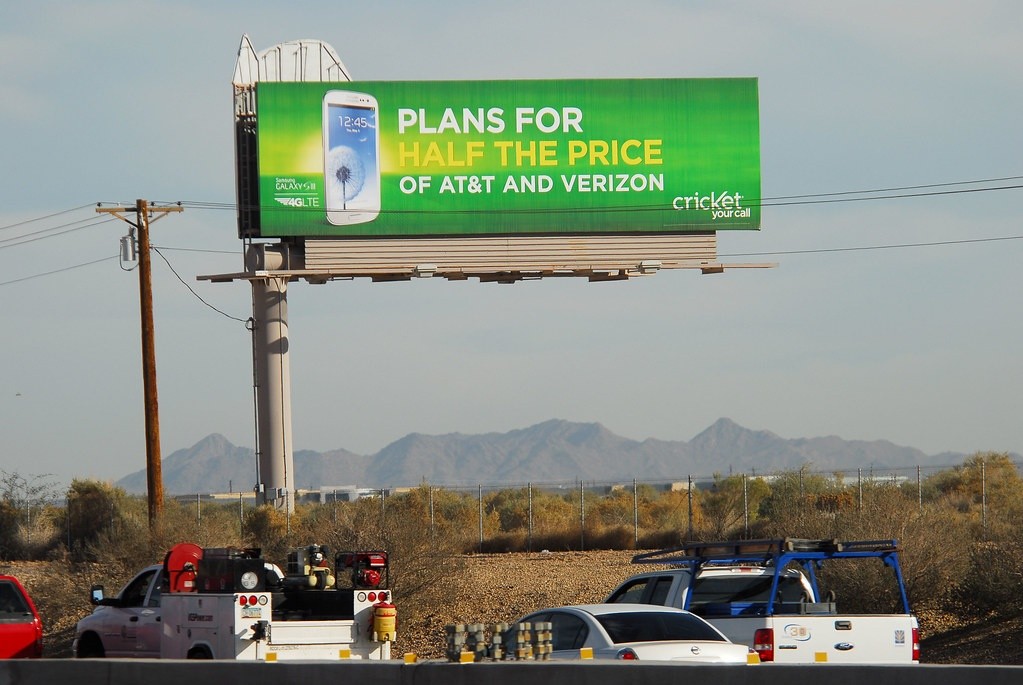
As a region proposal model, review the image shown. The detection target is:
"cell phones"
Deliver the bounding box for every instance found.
[323,90,381,224]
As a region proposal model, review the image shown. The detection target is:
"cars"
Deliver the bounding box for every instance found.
[0,573,43,659]
[474,601,762,667]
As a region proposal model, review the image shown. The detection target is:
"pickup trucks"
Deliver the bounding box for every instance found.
[69,560,392,660]
[598,538,919,666]
